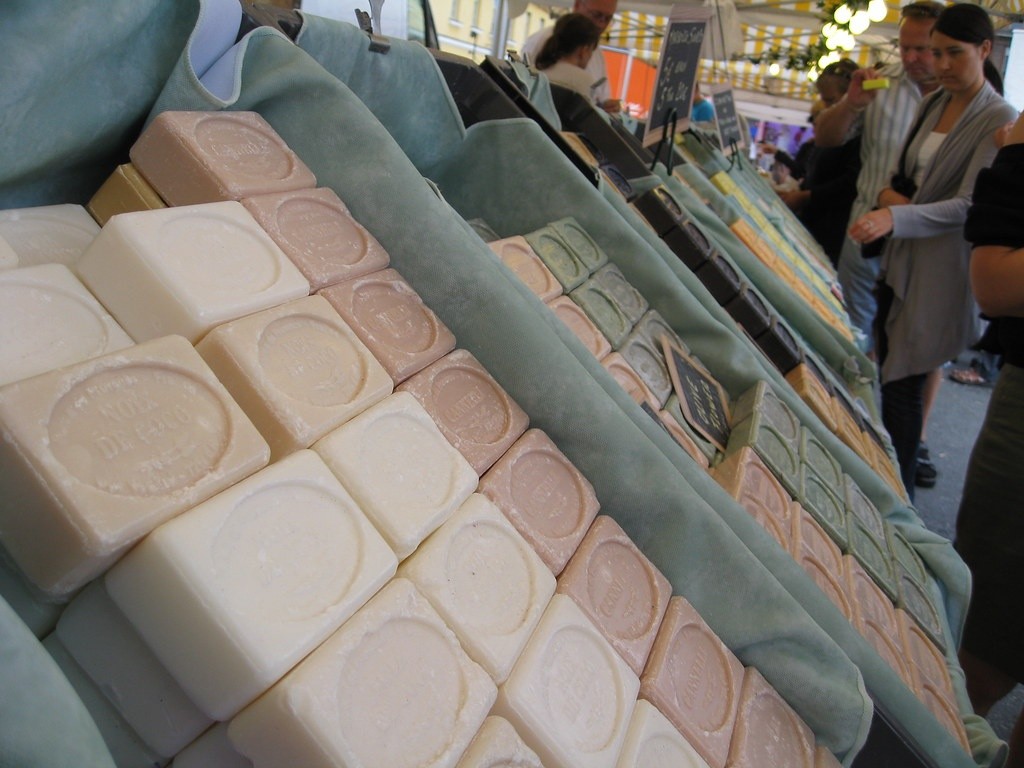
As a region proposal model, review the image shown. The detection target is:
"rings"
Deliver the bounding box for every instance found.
[866,219,874,228]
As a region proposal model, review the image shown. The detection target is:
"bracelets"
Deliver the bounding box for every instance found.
[843,95,866,114]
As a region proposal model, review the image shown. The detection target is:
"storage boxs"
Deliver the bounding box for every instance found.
[1,0,973,768]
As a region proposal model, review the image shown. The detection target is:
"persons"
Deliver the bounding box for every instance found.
[951,109,1024,768]
[848,3,1019,504]
[762,0,938,489]
[690,81,715,121]
[519,0,622,114]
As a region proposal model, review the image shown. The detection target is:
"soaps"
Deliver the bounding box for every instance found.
[0,108,977,768]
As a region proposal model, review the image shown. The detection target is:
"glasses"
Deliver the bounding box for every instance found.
[580,3,615,27]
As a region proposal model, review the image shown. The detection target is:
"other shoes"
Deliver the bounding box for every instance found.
[915,442,939,486]
[949,368,998,386]
